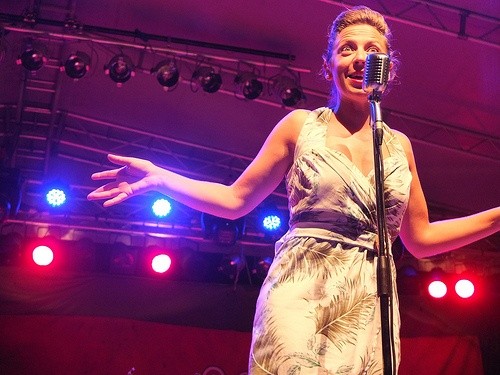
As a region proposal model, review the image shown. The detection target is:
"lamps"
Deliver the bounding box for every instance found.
[18,32,46,76]
[29,240,58,267]
[191,62,224,96]
[59,48,91,80]
[425,272,477,299]
[45,180,72,209]
[106,54,137,87]
[148,58,181,92]
[232,69,265,100]
[270,73,303,107]
[150,192,176,222]
[258,203,282,231]
[143,247,171,274]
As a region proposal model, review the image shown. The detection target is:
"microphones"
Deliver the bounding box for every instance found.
[362,51,391,145]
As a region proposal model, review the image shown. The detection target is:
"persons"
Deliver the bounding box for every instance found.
[84,5,499,375]
